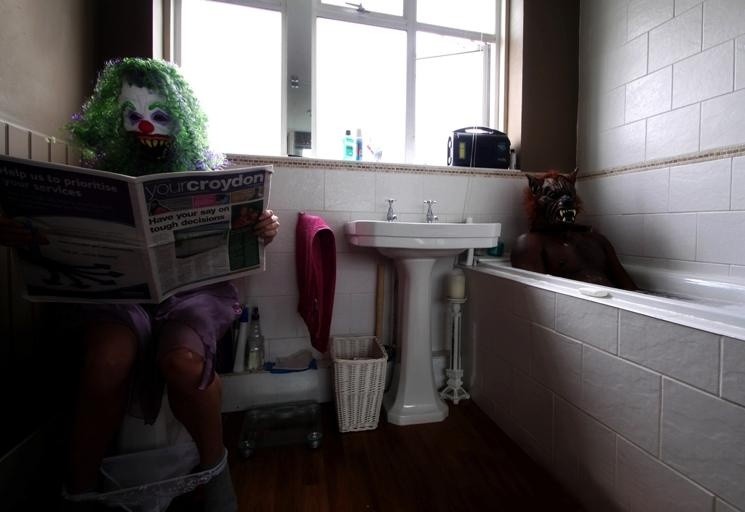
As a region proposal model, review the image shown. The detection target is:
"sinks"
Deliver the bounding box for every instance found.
[345,219,503,257]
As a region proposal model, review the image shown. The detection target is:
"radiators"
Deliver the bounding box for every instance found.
[0,119,91,171]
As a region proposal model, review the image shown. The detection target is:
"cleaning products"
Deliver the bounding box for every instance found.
[232,306,247,374]
[343,129,363,159]
[248,307,263,372]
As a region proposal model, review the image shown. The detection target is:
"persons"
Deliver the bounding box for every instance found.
[509,165,637,292]
[0,53,280,511]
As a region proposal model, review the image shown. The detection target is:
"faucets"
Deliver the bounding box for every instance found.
[425,199,440,222]
[385,197,398,220]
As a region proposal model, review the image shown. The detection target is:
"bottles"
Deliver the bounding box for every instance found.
[342,129,364,161]
[232,304,266,374]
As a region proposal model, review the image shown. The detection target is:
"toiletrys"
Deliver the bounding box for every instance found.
[490,239,506,259]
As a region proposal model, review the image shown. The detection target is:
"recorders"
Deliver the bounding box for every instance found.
[446,124,512,170]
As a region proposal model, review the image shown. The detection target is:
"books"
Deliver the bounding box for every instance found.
[0,152,275,306]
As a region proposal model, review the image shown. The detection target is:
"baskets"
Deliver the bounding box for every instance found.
[333,335,389,434]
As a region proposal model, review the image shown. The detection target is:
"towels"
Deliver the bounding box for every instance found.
[295,213,335,356]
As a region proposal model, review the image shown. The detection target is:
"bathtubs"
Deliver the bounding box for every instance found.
[478,256,745,326]
